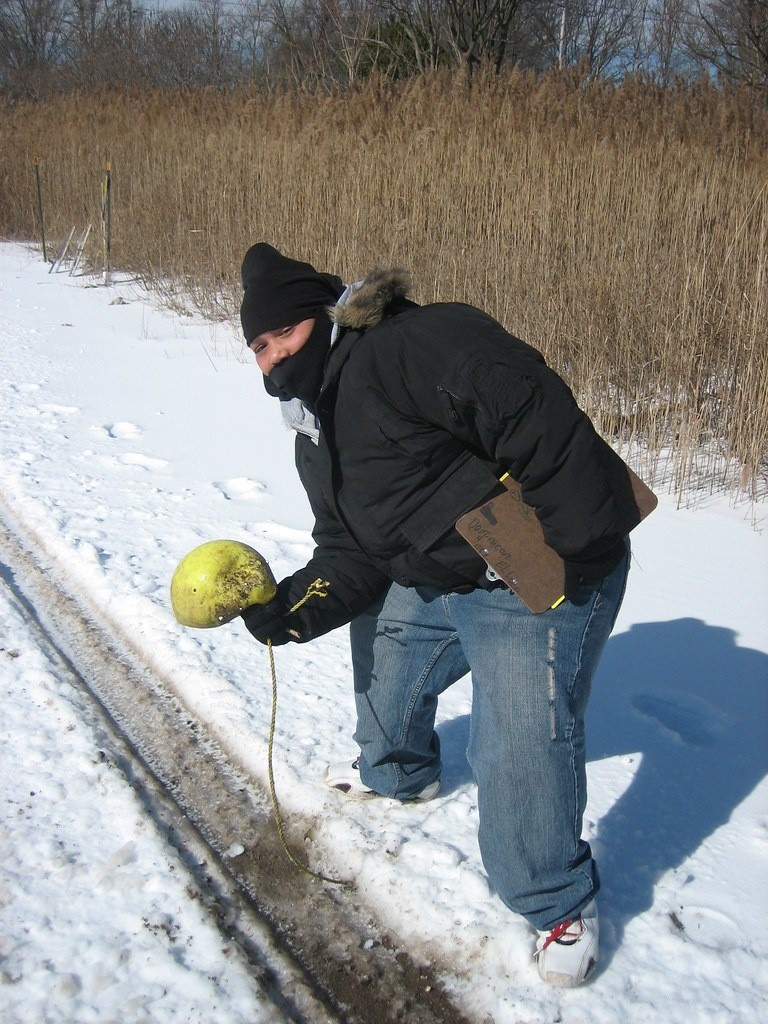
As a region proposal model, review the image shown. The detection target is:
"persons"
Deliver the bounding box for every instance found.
[240,241,658,988]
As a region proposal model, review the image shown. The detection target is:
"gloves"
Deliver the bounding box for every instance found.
[560,536,592,605]
[238,598,292,646]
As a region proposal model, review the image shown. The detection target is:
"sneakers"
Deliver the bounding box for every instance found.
[532,896,599,986]
[322,756,440,803]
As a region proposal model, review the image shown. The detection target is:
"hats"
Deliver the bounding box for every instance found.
[239,242,345,403]
